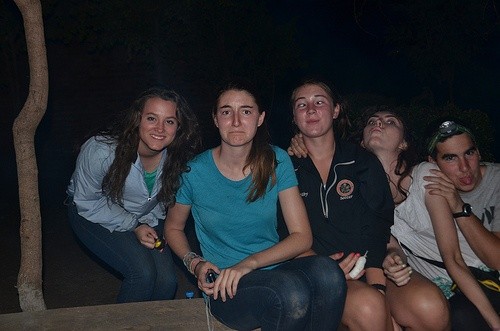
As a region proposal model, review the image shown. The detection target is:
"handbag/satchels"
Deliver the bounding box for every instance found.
[450,265,500,310]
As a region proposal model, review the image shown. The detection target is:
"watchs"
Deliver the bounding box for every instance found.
[453,203,473,218]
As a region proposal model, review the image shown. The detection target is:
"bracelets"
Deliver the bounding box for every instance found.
[369,283,387,293]
[182,251,207,279]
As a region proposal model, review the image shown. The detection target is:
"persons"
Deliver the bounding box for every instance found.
[62,86,205,304]
[161,78,348,331]
[277,75,500,331]
